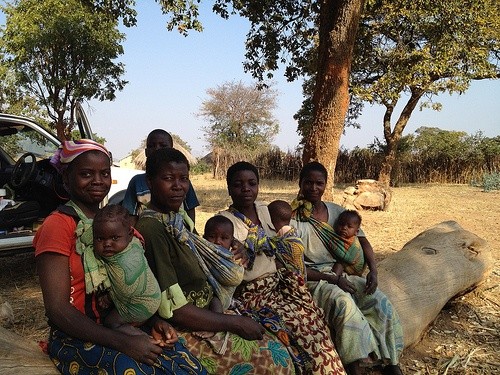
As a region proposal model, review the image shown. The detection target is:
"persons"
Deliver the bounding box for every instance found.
[33,140,210,375]
[329,209,366,276]
[289,162,404,375]
[136,148,299,375]
[216,161,348,375]
[192,213,245,338]
[119,129,201,234]
[267,199,305,279]
[92,205,162,341]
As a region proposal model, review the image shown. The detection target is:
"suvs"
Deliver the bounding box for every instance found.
[0,112,151,257]
